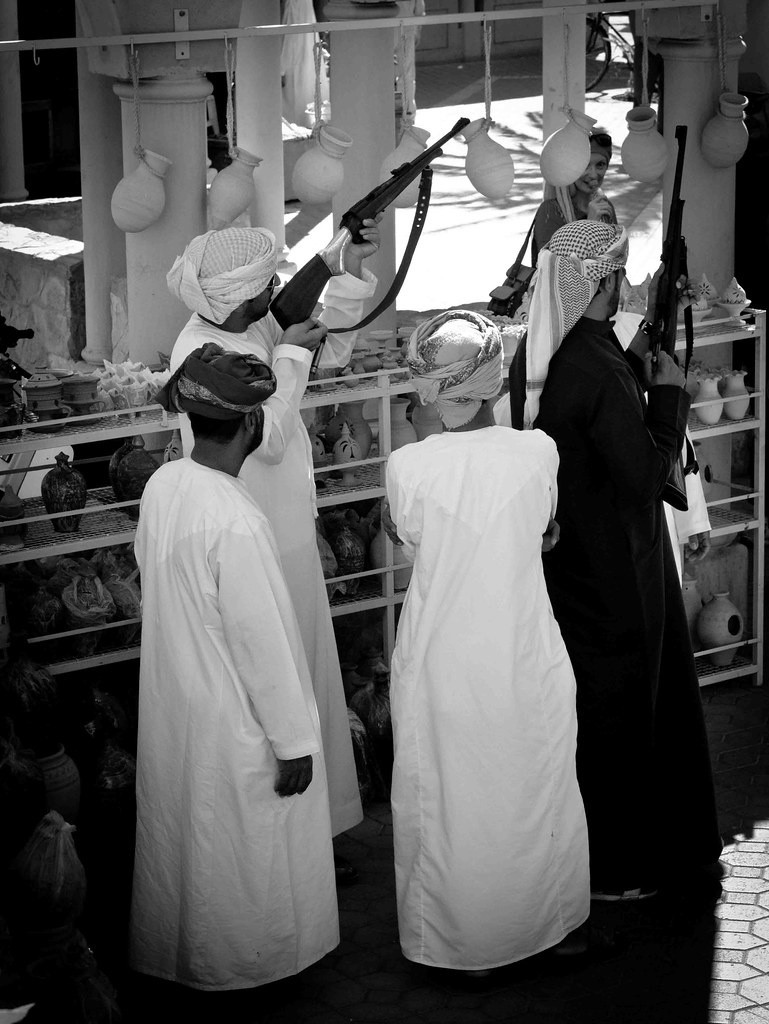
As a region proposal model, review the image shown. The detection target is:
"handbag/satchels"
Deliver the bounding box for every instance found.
[487,261,538,318]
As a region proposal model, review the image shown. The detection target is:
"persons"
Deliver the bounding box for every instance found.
[168,214,383,840]
[532,134,617,269]
[510,220,714,901]
[383,309,590,971]
[129,343,340,991]
[394,0,425,119]
[628,10,665,135]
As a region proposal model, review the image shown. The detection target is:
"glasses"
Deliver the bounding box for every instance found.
[589,133,612,149]
[266,274,275,294]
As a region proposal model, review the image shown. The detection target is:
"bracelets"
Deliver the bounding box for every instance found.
[638,320,652,335]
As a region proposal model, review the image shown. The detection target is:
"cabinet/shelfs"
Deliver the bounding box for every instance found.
[0,301,765,686]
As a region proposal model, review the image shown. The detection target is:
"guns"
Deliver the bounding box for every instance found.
[648,125,689,512]
[269,117,471,331]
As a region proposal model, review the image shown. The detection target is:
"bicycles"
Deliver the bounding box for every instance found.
[586,12,661,103]
